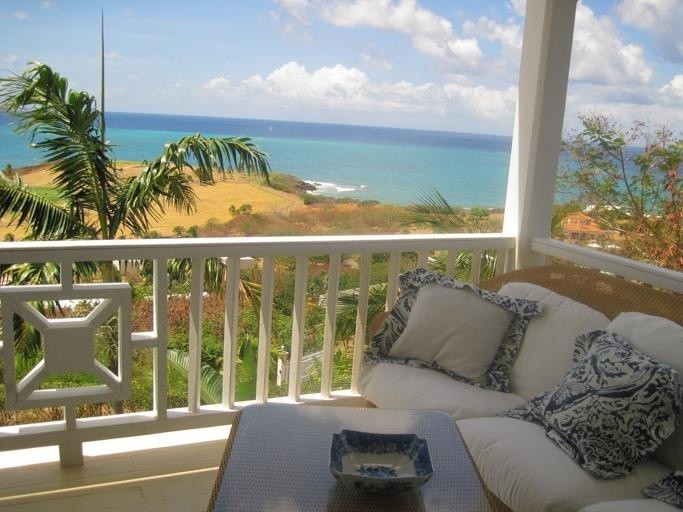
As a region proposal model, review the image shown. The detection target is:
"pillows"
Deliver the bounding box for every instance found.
[366,268,544,393]
[505,328,683,478]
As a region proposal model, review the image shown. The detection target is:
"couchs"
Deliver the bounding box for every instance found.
[357,264,683,512]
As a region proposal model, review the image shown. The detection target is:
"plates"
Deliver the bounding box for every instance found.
[328,428,436,495]
